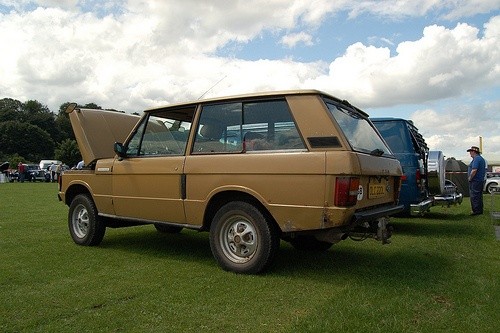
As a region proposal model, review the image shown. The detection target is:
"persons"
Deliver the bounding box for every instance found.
[466,146,486,216]
[50,162,56,183]
[18,162,25,182]
[0,162,12,184]
[55,162,61,183]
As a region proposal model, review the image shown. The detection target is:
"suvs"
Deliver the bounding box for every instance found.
[367,117,463,216]
[57,89,406,274]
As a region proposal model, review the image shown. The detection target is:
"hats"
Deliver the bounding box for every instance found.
[466,146,482,155]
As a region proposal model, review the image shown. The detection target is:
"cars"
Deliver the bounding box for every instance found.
[484,172,500,195]
[444,178,459,195]
[9,164,48,183]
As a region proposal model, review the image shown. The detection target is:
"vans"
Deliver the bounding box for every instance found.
[39,159,62,181]
